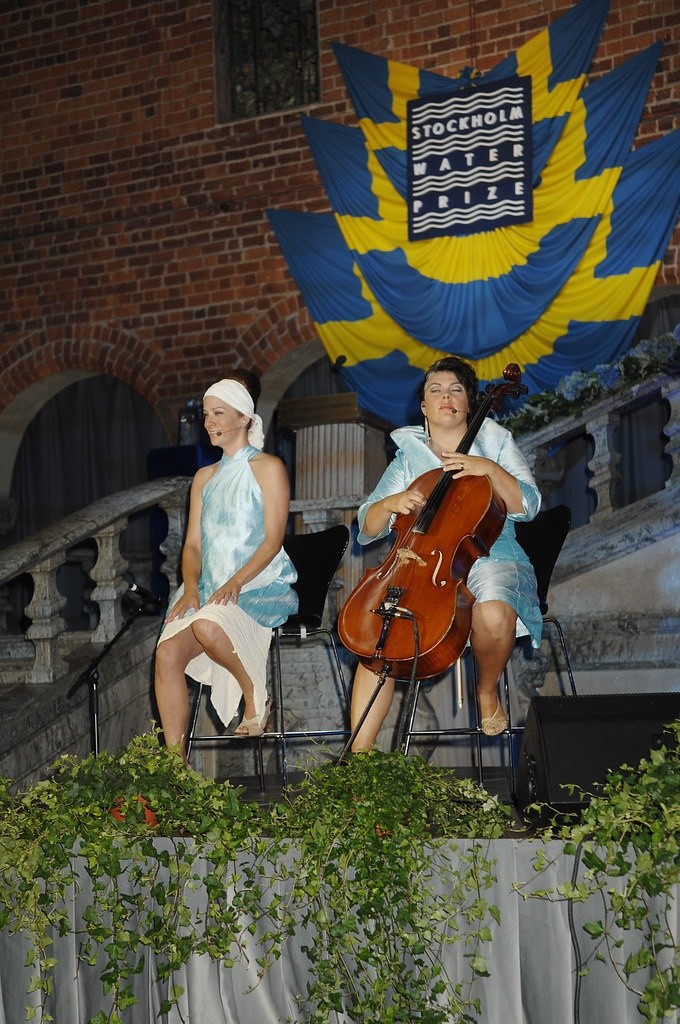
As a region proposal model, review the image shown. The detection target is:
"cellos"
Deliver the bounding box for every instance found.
[338,363,529,760]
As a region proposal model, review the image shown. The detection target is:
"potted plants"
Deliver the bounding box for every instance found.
[2,720,680,1024]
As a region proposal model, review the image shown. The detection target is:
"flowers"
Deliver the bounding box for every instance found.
[554,323,680,402]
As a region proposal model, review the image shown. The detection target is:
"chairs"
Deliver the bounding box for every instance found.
[401,503,578,799]
[187,524,351,800]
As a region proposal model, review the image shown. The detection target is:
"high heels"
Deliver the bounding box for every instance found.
[481,694,509,735]
[232,699,274,735]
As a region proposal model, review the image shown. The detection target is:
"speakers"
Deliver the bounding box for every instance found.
[514,691,680,828]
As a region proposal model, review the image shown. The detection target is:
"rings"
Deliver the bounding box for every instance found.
[461,463,464,469]
[227,592,231,594]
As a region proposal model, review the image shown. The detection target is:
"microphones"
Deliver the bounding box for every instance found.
[452,409,469,414]
[332,355,356,391]
[128,583,169,610]
[217,426,247,436]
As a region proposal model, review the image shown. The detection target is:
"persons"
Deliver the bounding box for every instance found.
[351,356,543,753]
[155,369,300,767]
